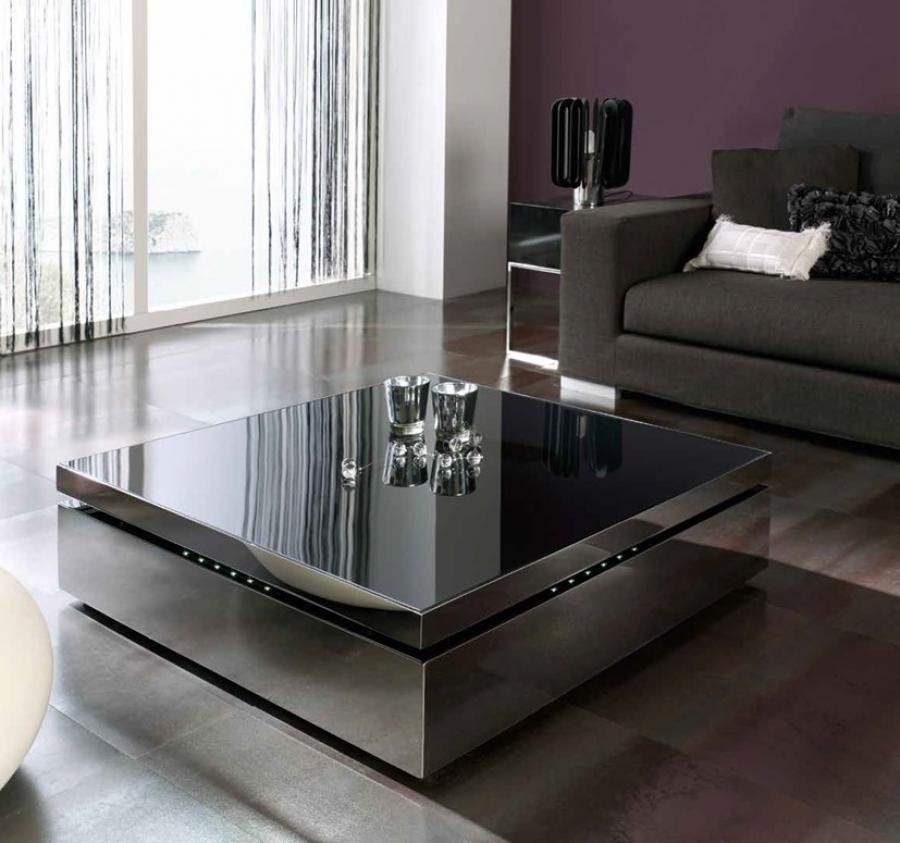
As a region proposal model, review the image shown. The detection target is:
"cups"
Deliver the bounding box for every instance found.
[429,438,477,497]
[383,375,430,436]
[431,382,479,441]
[381,433,428,487]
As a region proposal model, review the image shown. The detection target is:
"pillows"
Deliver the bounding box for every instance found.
[681,213,833,281]
[710,144,859,232]
[786,182,900,281]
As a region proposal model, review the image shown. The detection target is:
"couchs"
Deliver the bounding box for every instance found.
[560,107,900,450]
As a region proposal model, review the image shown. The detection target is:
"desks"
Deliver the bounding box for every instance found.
[56,373,775,779]
